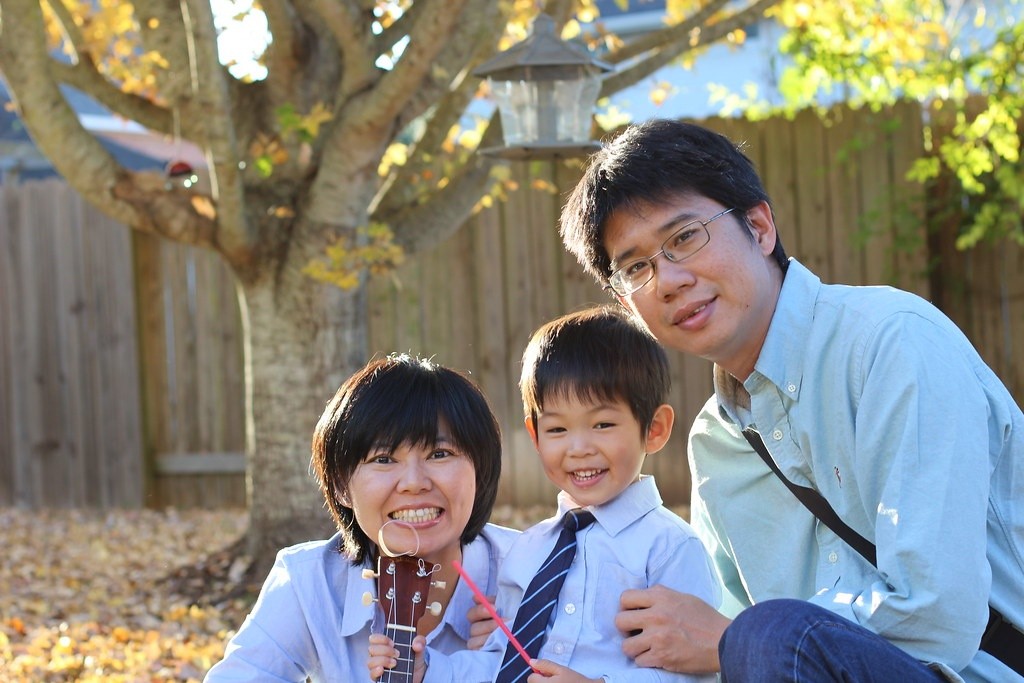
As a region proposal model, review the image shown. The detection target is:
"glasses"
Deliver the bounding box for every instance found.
[608,208,736,296]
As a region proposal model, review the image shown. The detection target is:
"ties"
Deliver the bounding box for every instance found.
[495,508,598,683]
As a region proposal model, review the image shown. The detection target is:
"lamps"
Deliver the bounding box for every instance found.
[471,13,614,147]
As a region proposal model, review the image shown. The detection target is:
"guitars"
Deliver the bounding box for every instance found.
[359,553,446,683]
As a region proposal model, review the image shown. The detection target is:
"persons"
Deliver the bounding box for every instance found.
[204,352,526,683]
[369,308,722,683]
[562,121,1024,683]
